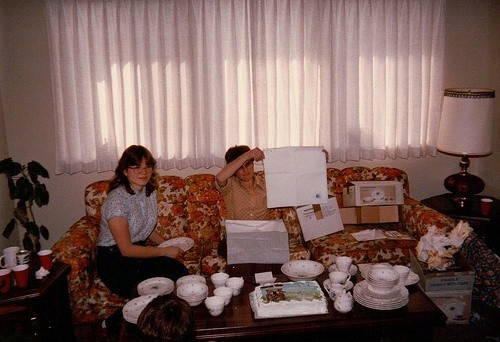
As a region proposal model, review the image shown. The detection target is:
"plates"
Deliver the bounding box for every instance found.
[355,281,409,310]
[176,274,206,284]
[158,237,195,252]
[123,276,174,326]
[330,265,357,276]
[403,272,419,286]
[323,279,354,291]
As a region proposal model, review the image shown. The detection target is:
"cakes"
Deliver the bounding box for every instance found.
[254,280,328,317]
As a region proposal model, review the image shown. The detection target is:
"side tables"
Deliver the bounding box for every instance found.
[0,255,76,342]
[421,192,500,258]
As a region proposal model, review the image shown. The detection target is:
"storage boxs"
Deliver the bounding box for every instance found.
[295,195,345,242]
[224,218,290,265]
[342,179,405,207]
[408,248,475,326]
[339,205,400,224]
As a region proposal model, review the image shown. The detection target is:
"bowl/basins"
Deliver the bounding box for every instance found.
[178,284,209,307]
[281,260,325,281]
[366,264,410,289]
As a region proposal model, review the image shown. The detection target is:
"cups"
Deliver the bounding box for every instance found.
[329,271,351,285]
[0,269,11,292]
[15,250,32,268]
[205,272,244,316]
[37,250,52,271]
[11,265,30,288]
[481,198,493,216]
[0,246,20,268]
[336,257,353,272]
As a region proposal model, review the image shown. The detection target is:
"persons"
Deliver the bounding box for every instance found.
[95,145,190,302]
[214,145,330,275]
[138,294,198,342]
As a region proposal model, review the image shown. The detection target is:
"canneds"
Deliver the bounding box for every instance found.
[16,249,30,266]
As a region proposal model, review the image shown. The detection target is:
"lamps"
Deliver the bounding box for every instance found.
[435,88,497,207]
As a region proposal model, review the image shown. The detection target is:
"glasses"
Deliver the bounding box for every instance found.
[129,165,153,174]
[235,161,254,170]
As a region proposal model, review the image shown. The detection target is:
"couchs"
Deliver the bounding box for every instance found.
[50,167,458,342]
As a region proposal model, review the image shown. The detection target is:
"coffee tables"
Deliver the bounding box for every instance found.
[119,273,450,342]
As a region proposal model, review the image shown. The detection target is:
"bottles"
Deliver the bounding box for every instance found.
[333,290,354,313]
